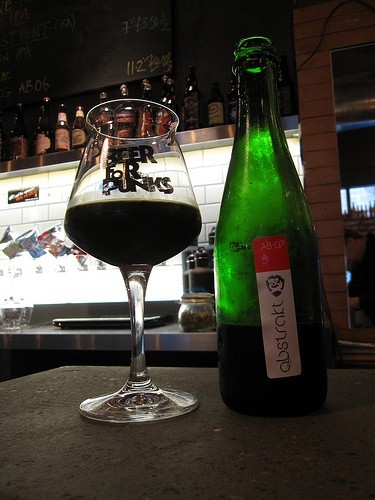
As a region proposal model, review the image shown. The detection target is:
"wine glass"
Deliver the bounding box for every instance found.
[64,98,202,424]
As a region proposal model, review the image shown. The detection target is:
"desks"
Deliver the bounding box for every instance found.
[0,365,375,500]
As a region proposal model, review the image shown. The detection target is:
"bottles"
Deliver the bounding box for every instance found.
[213,36,328,416]
[183,65,204,130]
[99,75,179,137]
[71,105,87,148]
[277,54,295,116]
[35,97,52,155]
[209,81,225,127]
[227,76,238,124]
[56,104,70,152]
[178,292,215,333]
[10,103,29,159]
